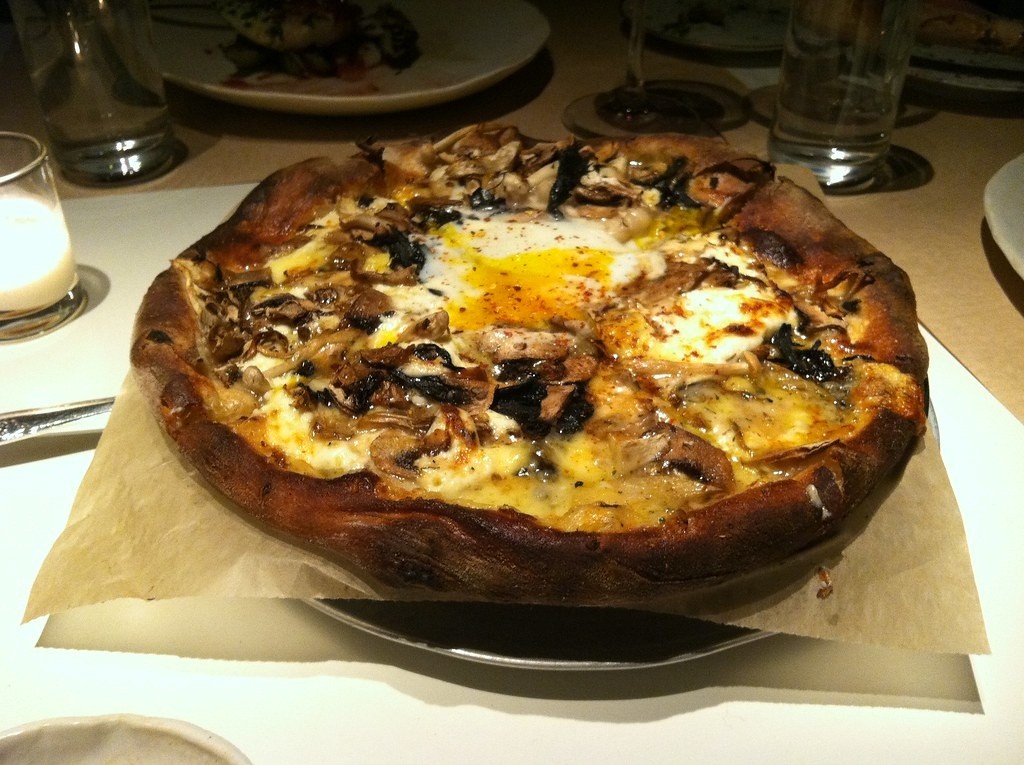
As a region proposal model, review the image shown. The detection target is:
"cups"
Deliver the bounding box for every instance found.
[12,1,178,187]
[768,0,916,186]
[0,131,84,341]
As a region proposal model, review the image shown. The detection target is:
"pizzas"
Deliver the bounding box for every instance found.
[131,127,933,606]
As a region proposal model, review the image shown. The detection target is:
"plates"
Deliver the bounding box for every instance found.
[151,0,551,115]
[302,594,783,673]
[983,152,1024,282]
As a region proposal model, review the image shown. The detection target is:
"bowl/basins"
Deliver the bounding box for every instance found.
[0,713,253,765]
